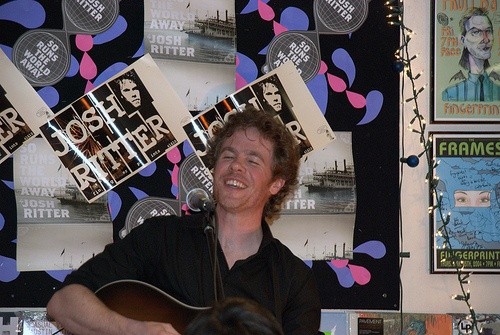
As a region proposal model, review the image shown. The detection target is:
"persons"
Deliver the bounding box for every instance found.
[185,298,284,335]
[46,108,326,335]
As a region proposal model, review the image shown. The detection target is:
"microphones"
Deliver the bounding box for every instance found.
[186,188,216,213]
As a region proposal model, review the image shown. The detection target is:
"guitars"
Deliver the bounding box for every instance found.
[94,278,213,335]
